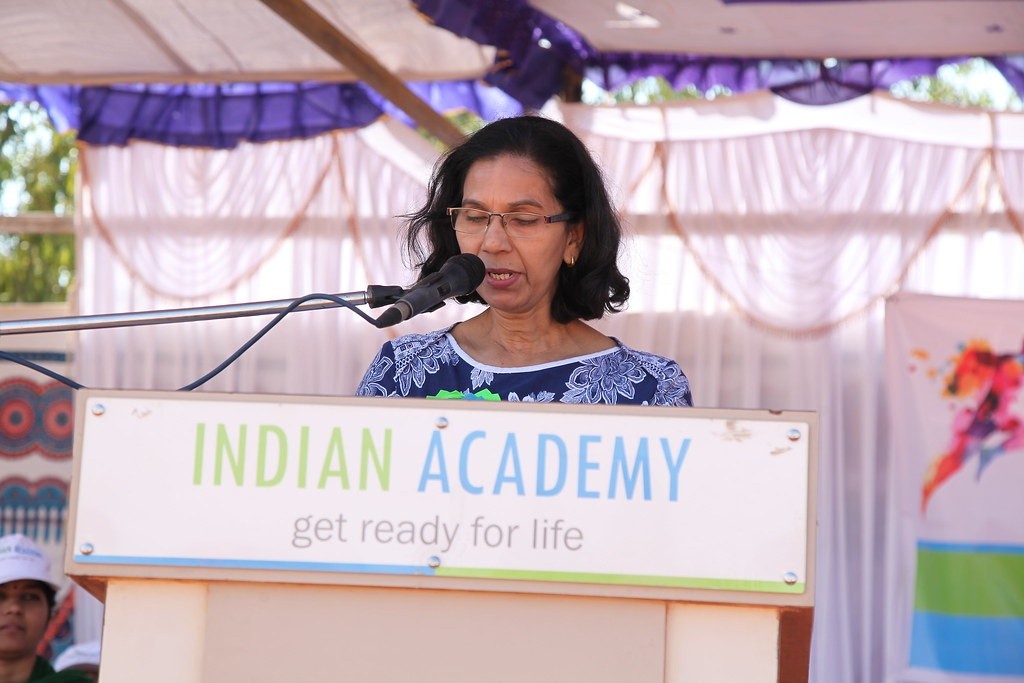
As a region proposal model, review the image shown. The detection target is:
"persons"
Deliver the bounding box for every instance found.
[354,117,694,408]
[0,533,95,683]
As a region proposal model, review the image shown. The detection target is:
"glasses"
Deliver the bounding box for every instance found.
[446,207,571,238]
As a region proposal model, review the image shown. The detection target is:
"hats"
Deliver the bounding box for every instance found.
[0,534,60,591]
[54,643,101,674]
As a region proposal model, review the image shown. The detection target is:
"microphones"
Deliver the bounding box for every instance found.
[375,253,486,329]
